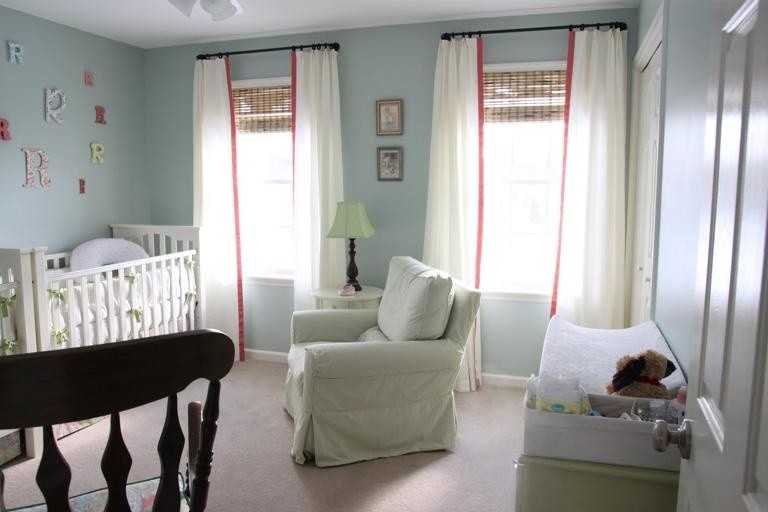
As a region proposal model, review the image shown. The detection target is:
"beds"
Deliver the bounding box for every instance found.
[0,222,204,370]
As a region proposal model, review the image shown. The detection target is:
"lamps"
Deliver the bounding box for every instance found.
[321,200,378,292]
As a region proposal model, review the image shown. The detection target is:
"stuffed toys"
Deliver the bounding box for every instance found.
[606,350,677,399]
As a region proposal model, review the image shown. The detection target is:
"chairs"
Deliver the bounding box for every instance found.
[286,252,483,468]
[0,327,240,512]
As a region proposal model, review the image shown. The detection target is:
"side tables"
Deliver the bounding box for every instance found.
[311,284,383,311]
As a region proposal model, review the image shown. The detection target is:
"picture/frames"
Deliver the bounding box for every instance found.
[376,147,403,181]
[376,99,402,135]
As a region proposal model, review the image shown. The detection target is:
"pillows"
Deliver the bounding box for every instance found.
[349,253,453,349]
